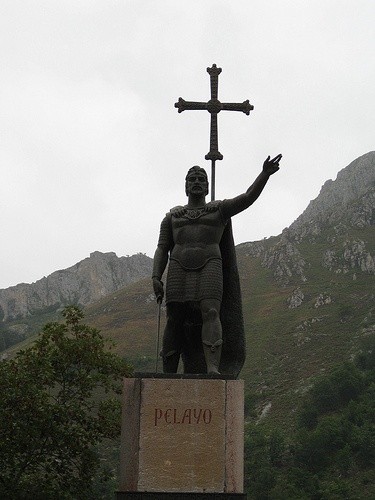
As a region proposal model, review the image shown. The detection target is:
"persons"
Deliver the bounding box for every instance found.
[150,154,283,378]
